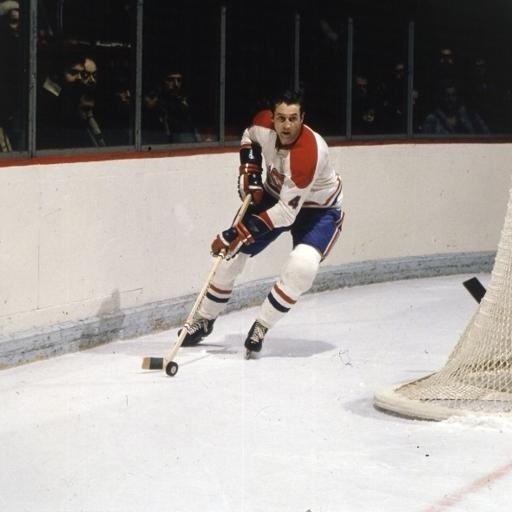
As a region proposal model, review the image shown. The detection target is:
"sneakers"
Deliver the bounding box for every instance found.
[178,318,215,343]
[245,322,267,351]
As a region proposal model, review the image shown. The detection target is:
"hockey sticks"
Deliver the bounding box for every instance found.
[142,193,253,371]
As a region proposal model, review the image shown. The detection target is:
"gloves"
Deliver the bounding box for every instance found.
[238,163,264,205]
[212,222,254,261]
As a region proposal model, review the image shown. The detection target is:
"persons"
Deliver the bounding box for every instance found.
[178,87,344,352]
[0,0,201,150]
[330,49,492,135]
